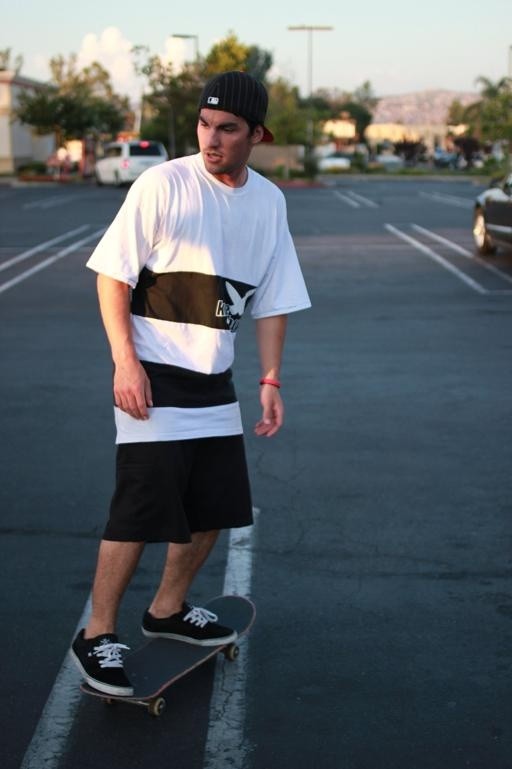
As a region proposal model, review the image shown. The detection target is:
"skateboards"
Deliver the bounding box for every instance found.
[80,594,255,715]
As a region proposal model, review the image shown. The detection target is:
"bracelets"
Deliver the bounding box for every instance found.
[258,379,281,388]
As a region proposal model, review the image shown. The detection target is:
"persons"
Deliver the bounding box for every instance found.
[66,71,314,697]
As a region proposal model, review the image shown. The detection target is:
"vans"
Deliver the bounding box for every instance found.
[93,141,170,185]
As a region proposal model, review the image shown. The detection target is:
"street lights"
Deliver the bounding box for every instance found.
[284,26,340,180]
[171,30,203,63]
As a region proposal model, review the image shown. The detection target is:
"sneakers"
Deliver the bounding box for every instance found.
[69,627,136,698]
[140,598,239,647]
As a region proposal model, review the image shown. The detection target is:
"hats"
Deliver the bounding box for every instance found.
[199,71,274,143]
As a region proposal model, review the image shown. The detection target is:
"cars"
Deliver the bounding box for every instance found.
[320,142,489,174]
[15,158,81,179]
[471,171,512,261]
